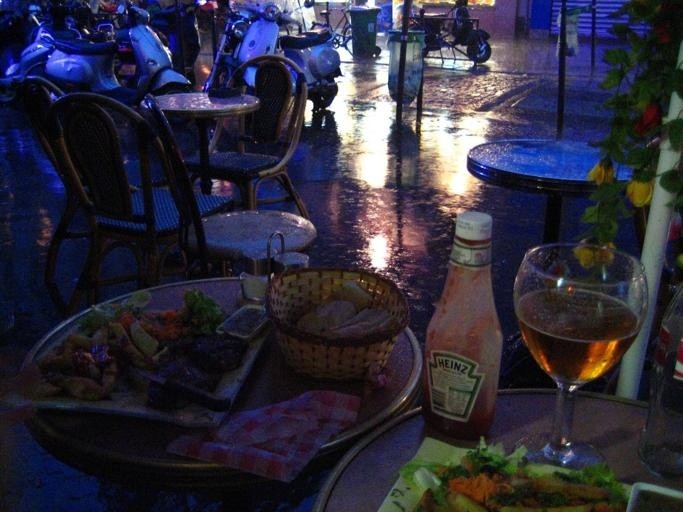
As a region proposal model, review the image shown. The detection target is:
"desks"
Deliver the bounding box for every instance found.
[468,137,632,244]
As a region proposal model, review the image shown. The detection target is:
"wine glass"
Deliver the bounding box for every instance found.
[513,242,647,469]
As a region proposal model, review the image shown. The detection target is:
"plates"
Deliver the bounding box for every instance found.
[215,301,270,344]
[2,303,272,429]
[377,439,636,512]
[626,482,682,512]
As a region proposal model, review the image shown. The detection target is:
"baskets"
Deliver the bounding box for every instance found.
[266,230,411,383]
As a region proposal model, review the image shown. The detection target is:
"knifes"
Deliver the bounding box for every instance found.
[129,366,231,415]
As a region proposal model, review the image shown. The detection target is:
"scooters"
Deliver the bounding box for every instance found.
[0,0,197,133]
[203,0,344,119]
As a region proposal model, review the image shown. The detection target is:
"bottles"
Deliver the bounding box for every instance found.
[638,274,683,481]
[421,208,503,435]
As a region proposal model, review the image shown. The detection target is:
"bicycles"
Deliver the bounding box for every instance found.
[309,7,371,59]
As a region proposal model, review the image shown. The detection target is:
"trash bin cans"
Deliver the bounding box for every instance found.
[347,7,381,57]
[386,29,426,107]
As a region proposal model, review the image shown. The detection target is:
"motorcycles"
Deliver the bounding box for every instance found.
[399,1,493,64]
